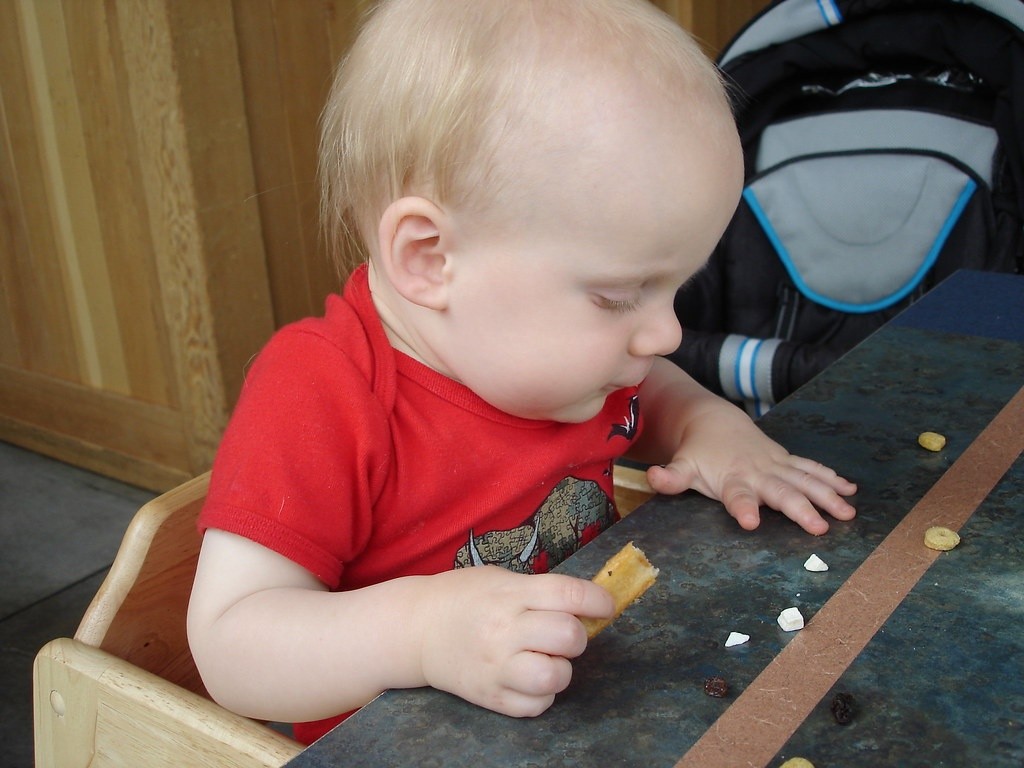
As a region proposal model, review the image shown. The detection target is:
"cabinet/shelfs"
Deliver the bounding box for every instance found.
[0,0,776,495]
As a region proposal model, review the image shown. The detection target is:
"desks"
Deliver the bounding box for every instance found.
[279,265,1024,768]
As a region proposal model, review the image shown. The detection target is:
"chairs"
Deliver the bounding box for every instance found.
[34,469,314,768]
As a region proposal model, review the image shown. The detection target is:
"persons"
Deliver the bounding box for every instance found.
[186,0,859,746]
